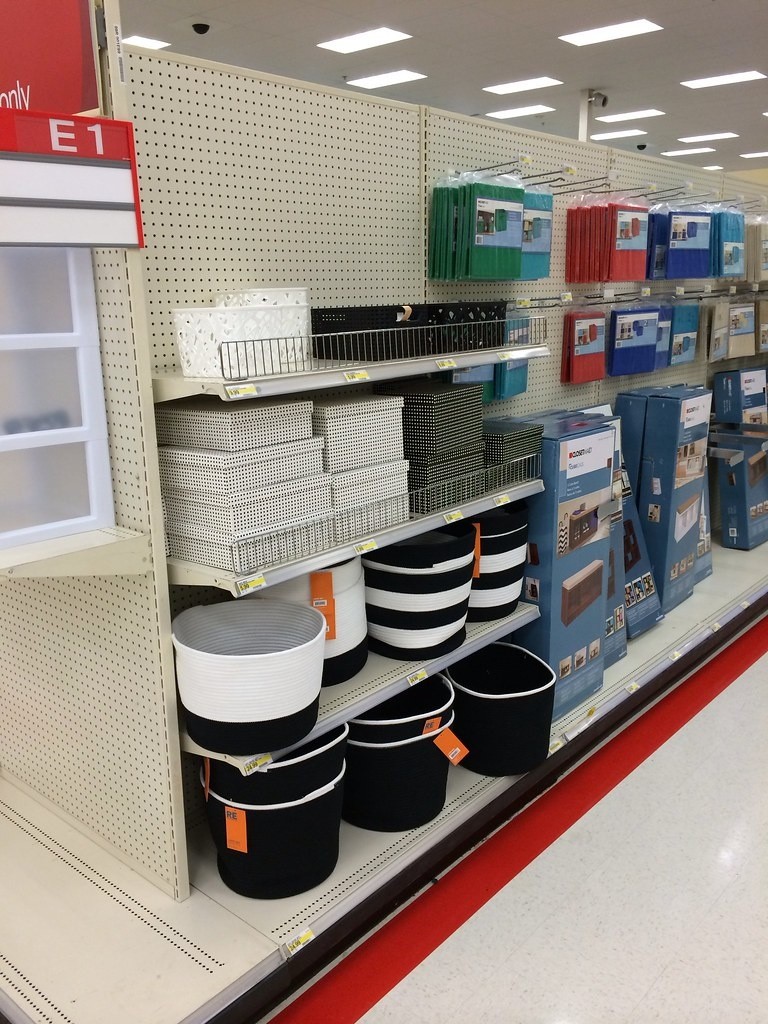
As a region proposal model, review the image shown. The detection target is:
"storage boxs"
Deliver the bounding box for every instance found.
[156,366,768,727]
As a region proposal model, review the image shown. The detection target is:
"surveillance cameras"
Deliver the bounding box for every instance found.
[637,145,646,150]
[192,23,210,34]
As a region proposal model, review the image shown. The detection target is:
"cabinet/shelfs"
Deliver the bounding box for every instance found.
[0,248,114,548]
[150,343,554,772]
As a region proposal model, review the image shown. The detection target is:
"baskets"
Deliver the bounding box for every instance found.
[216,288,308,364]
[172,304,311,380]
